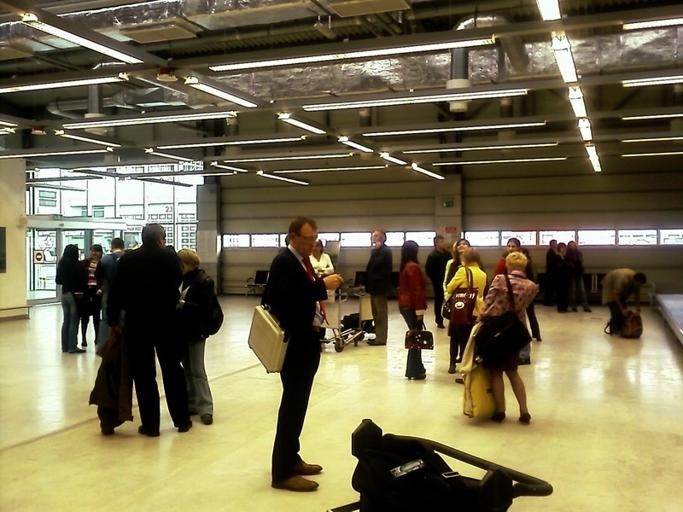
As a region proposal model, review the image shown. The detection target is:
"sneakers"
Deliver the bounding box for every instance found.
[179,420,192,432]
[139,426,160,437]
[102,427,115,435]
[449,367,456,373]
[202,414,213,424]
[69,348,86,353]
[82,340,87,346]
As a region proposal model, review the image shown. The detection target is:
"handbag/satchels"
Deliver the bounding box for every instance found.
[476,310,532,364]
[352,419,471,512]
[450,282,479,326]
[247,304,290,373]
[405,320,433,349]
[463,364,496,418]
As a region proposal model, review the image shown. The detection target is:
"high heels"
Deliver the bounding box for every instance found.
[404,368,426,377]
[408,373,426,380]
[492,412,505,423]
[519,413,531,424]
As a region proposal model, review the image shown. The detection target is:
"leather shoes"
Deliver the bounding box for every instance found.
[295,458,322,475]
[272,474,319,491]
[368,339,376,341]
[367,341,386,345]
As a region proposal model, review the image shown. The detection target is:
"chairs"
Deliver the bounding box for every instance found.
[391,272,400,294]
[245,270,269,297]
[347,272,368,297]
[530,271,605,305]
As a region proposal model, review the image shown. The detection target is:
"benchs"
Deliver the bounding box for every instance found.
[0,306,32,319]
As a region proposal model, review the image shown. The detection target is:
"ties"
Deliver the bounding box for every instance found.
[302,258,330,326]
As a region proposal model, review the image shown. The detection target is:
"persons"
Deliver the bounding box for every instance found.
[309,239,335,340]
[55,223,213,437]
[600,268,647,336]
[398,241,428,379]
[365,230,393,345]
[424,235,592,424]
[260,215,344,492]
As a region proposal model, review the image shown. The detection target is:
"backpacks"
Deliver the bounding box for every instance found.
[622,311,643,339]
[196,274,224,338]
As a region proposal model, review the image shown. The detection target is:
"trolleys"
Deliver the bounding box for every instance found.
[325,418,554,512]
[321,286,365,354]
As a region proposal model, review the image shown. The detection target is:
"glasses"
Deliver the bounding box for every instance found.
[300,235,317,244]
